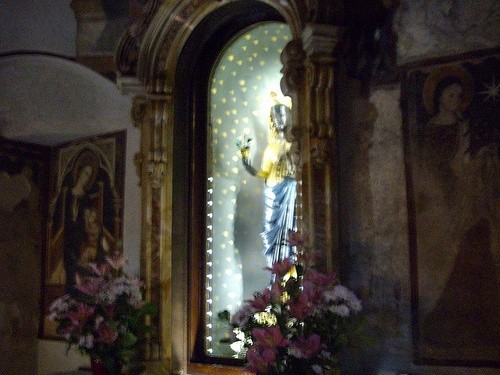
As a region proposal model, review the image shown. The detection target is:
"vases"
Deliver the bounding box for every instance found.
[90,354,124,375]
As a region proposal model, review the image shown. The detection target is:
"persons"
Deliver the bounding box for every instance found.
[236,103,298,295]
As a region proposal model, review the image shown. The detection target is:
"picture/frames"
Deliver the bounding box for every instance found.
[399,44,500,369]
[36,129,128,344]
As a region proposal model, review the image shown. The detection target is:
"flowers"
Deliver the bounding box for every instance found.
[217,229,363,375]
[48,247,161,372]
